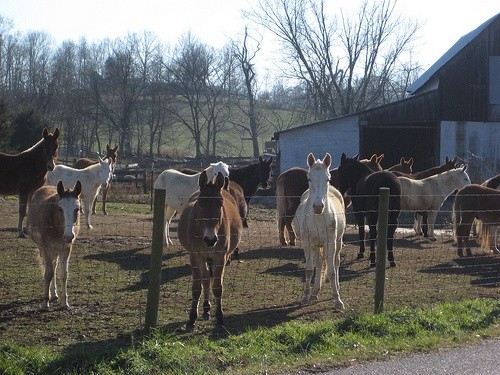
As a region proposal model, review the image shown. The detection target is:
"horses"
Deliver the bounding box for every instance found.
[150,155,274,339]
[275,152,500,312]
[0,128,120,312]
